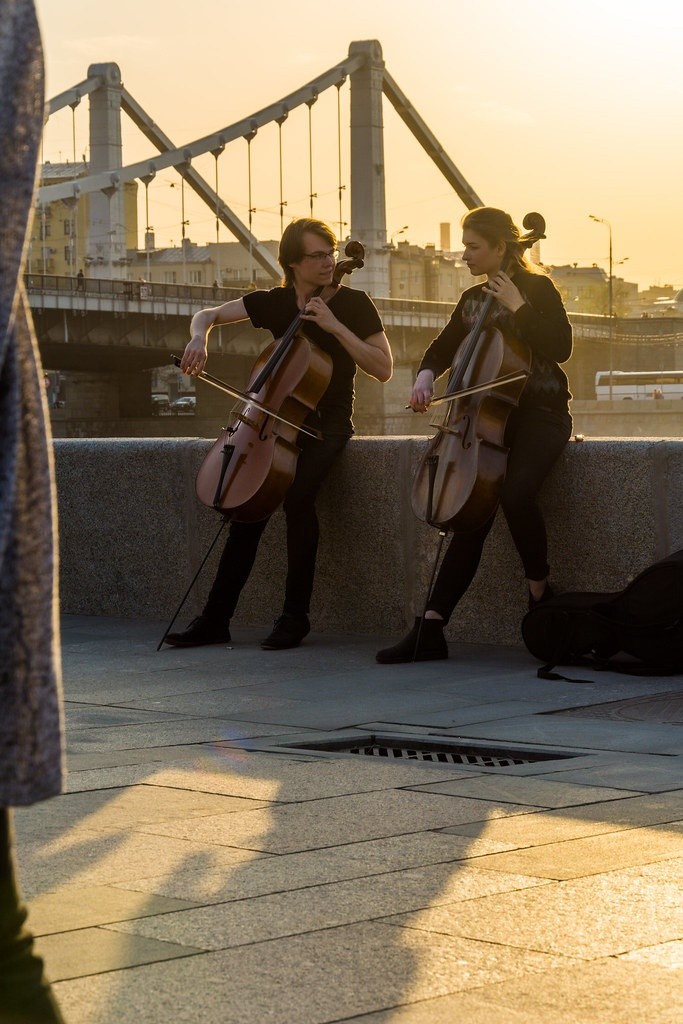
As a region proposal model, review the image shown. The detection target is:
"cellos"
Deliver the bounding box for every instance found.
[410,212,548,664]
[154,241,365,652]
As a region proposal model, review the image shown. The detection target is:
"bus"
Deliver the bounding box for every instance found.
[594,369,683,399]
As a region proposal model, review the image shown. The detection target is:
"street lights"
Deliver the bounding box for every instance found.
[588,214,615,401]
[391,226,409,245]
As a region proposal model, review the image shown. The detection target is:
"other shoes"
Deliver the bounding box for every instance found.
[261,614,311,650]
[164,616,232,646]
[376,616,448,664]
[529,581,551,610]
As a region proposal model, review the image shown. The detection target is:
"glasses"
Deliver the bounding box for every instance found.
[303,248,340,261]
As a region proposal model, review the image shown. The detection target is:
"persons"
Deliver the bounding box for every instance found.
[376,208,574,662]
[0,0,68,1024]
[162,217,394,650]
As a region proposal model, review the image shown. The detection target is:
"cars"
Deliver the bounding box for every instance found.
[168,396,197,413]
[151,394,169,413]
